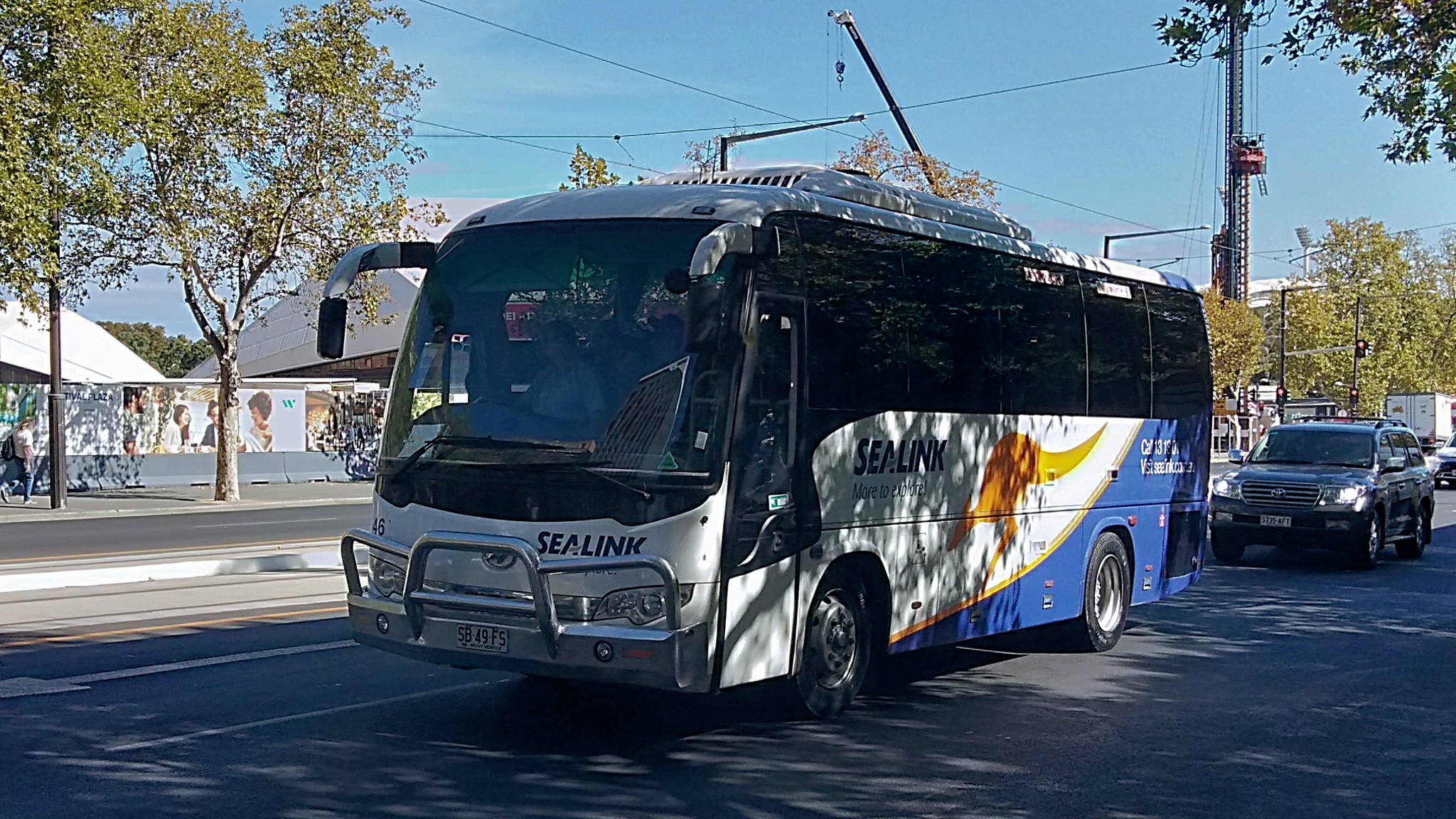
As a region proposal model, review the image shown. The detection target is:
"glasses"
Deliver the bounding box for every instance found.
[210,414,218,417]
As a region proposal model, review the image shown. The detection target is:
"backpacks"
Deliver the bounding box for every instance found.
[1,427,19,462]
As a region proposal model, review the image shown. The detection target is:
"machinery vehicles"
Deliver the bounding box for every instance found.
[827,7,948,197]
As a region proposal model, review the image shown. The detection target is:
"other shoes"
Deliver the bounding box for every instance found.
[23,500,38,504]
[1,489,9,503]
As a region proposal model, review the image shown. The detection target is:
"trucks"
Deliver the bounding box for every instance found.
[1385,391,1453,455]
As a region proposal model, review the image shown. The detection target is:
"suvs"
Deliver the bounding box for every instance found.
[1209,414,1435,569]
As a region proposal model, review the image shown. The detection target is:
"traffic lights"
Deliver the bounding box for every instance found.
[1349,388,1358,404]
[1278,409,1284,417]
[1259,404,1263,412]
[1355,340,1367,358]
[1275,387,1286,404]
[1249,389,1255,402]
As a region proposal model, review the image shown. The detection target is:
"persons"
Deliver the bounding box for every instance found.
[1258,424,1268,455]
[522,312,776,444]
[1,417,38,505]
[123,386,272,456]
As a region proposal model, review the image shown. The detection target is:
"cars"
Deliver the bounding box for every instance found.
[1431,431,1456,489]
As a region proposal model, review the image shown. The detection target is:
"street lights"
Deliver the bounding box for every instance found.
[720,112,866,171]
[1295,226,1312,279]
[1351,290,1436,415]
[1104,225,1212,262]
[1279,279,1371,425]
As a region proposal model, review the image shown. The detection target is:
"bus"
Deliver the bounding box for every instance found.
[317,163,1214,720]
